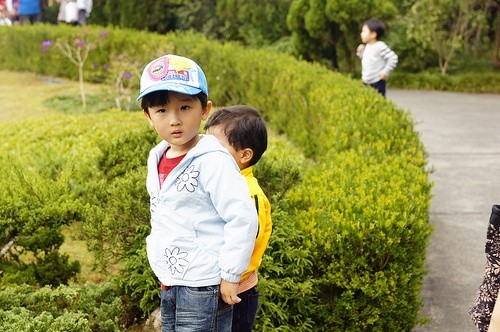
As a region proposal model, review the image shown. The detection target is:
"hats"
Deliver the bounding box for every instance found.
[136,54,208,101]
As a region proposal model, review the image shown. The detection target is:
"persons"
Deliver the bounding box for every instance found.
[204,105,273,332]
[136,55,259,332]
[356,19,398,99]
[0,0,93,27]
